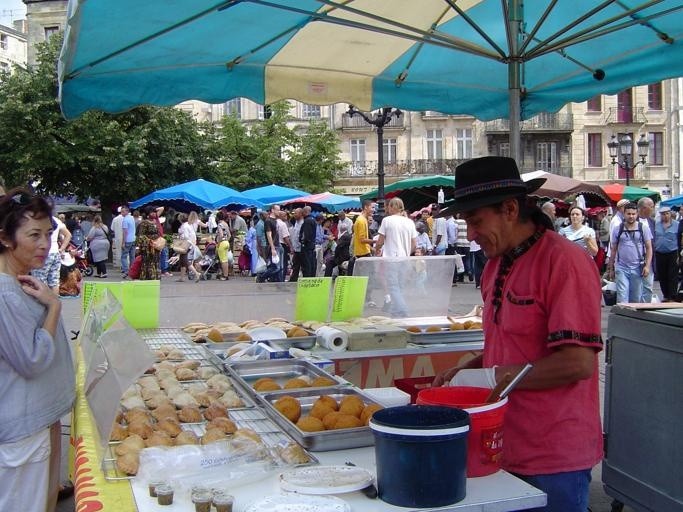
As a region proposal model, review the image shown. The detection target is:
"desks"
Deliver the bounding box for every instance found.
[73,363,548,507]
[71,314,488,359]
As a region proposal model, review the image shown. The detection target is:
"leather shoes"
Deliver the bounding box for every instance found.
[456,278,464,282]
[469,273,473,281]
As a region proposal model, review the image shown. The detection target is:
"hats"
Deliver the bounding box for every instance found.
[659,206,670,213]
[432,156,547,220]
[616,198,630,207]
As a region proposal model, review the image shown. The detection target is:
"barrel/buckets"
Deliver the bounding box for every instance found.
[368,404,472,509]
[416,383,510,478]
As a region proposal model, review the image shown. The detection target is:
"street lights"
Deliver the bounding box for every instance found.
[345,104,402,225]
[606,130,651,187]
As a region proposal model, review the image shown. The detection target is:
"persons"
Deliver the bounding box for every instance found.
[444,215,458,255]
[111,206,124,267]
[415,222,432,256]
[316,215,335,277]
[431,203,448,255]
[300,206,317,277]
[421,210,429,221]
[541,201,558,222]
[456,213,474,282]
[0,187,77,512]
[368,216,376,247]
[372,197,416,318]
[677,203,683,301]
[229,211,248,232]
[176,213,203,282]
[430,156,606,512]
[606,199,630,230]
[468,241,488,288]
[322,219,335,255]
[254,205,283,283]
[354,198,377,258]
[154,206,172,278]
[86,215,110,278]
[277,212,294,282]
[188,210,208,229]
[255,211,269,260]
[427,213,434,242]
[210,211,232,280]
[329,217,338,241]
[654,207,678,304]
[414,247,427,300]
[324,224,351,277]
[557,207,599,257]
[135,207,160,280]
[595,213,610,245]
[416,213,422,221]
[120,206,136,278]
[81,215,92,233]
[637,197,656,303]
[608,203,653,304]
[62,213,81,230]
[133,211,141,222]
[337,211,353,237]
[287,208,304,282]
[31,215,72,297]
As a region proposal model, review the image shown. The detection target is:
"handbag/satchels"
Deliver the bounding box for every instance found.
[99,224,112,243]
[172,232,191,254]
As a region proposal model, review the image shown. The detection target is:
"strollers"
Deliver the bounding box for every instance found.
[66,239,93,276]
[198,241,221,281]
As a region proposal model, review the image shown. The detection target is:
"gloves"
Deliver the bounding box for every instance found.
[448,365,498,393]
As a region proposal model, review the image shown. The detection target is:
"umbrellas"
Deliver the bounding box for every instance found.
[359,173,463,214]
[242,183,312,210]
[600,183,662,203]
[282,191,362,214]
[128,179,264,210]
[520,168,612,211]
[58,1,683,162]
[660,192,683,209]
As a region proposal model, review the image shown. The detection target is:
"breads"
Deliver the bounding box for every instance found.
[425,326,442,332]
[257,381,281,391]
[230,436,254,449]
[450,322,464,331]
[199,367,217,379]
[464,320,473,329]
[254,378,274,389]
[293,329,308,337]
[192,333,206,343]
[207,373,230,387]
[283,379,309,389]
[145,431,173,448]
[119,396,145,409]
[361,405,384,427]
[161,344,176,355]
[335,415,363,429]
[469,322,481,329]
[125,408,152,424]
[203,402,229,420]
[232,427,260,444]
[310,404,335,421]
[280,444,308,464]
[176,360,201,370]
[314,396,338,411]
[297,416,325,433]
[128,421,152,439]
[146,394,172,407]
[208,328,224,342]
[109,427,128,441]
[204,417,238,436]
[177,406,202,423]
[173,431,200,445]
[312,377,336,386]
[239,319,265,329]
[176,367,198,380]
[115,434,145,456]
[138,360,185,400]
[213,322,239,331]
[191,328,207,337]
[116,453,139,475]
[218,390,245,408]
[265,317,289,328]
[152,417,182,437]
[201,429,227,445]
[322,412,345,430]
[183,326,207,333]
[236,333,250,340]
[287,326,299,337]
[121,384,141,399]
[151,404,179,422]
[180,323,207,329]
[273,396,301,424]
[171,394,201,409]
[339,396,365,417]
[189,381,231,407]
[167,350,186,360]
[407,326,421,333]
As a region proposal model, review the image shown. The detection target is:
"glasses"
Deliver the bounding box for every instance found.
[11,192,31,205]
[625,205,637,209]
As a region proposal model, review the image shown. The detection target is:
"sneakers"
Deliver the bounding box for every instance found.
[175,277,185,282]
[100,274,107,278]
[57,478,73,500]
[195,273,202,283]
[217,276,228,280]
[93,274,101,276]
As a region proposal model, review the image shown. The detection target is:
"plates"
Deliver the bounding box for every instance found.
[201,341,270,373]
[280,466,375,494]
[395,324,485,343]
[243,493,350,512]
[257,384,389,451]
[205,335,251,343]
[252,324,317,350]
[225,358,342,398]
